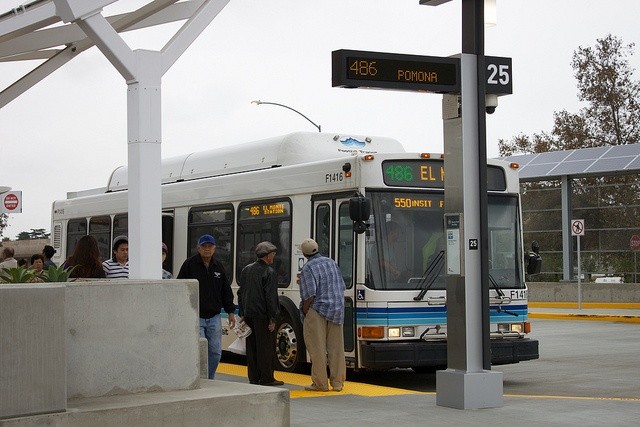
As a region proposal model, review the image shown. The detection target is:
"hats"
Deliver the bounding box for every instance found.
[301,238,318,254]
[255,241,277,257]
[113,235,128,246]
[198,234,216,246]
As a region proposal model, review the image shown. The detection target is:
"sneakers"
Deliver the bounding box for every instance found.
[333,383,343,391]
[259,379,284,385]
[304,382,329,391]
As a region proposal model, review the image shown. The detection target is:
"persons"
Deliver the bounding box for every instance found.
[59,234,105,277]
[237,240,284,385]
[42,245,57,269]
[299,238,346,391]
[424,237,445,274]
[176,234,236,378]
[370,220,401,289]
[162,242,172,278]
[102,235,129,278]
[0,246,17,282]
[30,254,45,282]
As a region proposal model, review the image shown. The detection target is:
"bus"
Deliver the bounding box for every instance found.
[51,132,538,377]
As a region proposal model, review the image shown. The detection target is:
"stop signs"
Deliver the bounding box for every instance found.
[630,235,640,250]
[0,190,22,212]
